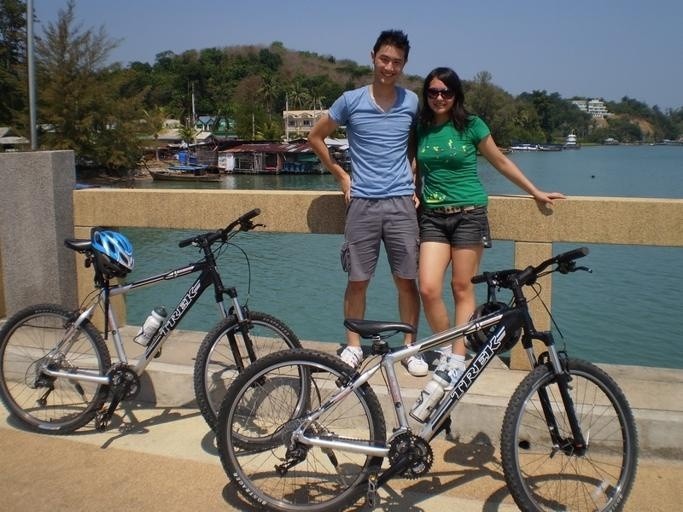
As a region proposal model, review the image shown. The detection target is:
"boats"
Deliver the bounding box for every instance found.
[653,137,682,146]
[562,132,581,151]
[604,138,620,146]
[144,165,225,184]
[495,144,564,154]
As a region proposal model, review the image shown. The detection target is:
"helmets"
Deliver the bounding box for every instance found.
[464,302,522,354]
[90,227,135,278]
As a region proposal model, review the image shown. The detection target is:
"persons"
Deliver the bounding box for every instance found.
[305,27,436,376]
[407,67,566,393]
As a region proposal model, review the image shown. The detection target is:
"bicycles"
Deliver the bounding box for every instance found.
[216,245,641,510]
[0,205,314,453]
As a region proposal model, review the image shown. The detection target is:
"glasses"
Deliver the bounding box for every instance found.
[426,88,454,100]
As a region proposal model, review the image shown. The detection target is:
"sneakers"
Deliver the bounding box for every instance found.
[400,343,429,376]
[338,346,364,368]
[432,350,466,390]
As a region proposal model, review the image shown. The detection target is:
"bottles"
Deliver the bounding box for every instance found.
[410,368,452,424]
[133,304,167,346]
